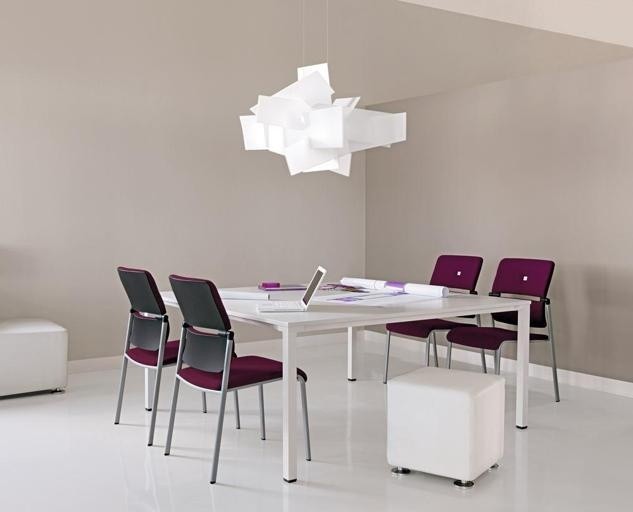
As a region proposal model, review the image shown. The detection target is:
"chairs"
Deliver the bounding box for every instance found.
[383,254,487,383]
[114,266,240,446]
[164,275,311,484]
[446,258,560,429]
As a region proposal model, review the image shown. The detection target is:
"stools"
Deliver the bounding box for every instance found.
[386,367,505,488]
[1,318,69,396]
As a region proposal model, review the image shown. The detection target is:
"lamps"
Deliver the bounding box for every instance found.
[239,63,407,178]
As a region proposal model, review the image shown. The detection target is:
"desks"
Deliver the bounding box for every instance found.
[159,283,532,482]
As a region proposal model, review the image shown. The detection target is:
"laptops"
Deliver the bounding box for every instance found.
[258,265,327,313]
[258,283,334,291]
